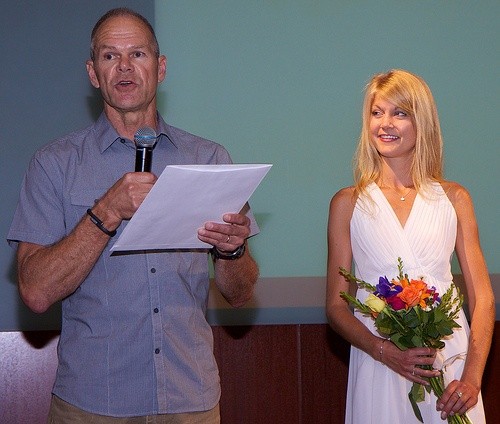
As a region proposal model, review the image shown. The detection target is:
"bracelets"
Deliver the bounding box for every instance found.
[86,208,117,237]
[379,339,388,365]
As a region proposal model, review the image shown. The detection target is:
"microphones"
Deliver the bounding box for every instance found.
[133,126,157,172]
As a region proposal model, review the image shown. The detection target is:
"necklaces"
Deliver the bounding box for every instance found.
[379,177,415,201]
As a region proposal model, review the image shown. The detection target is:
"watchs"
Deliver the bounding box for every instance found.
[212,243,246,260]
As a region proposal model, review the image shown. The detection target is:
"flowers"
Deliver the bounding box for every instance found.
[339,256,473,424]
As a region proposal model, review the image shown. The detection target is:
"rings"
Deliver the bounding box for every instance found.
[455,390,462,399]
[226,235,230,243]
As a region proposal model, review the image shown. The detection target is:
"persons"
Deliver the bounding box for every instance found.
[326,68,496,424]
[6,8,260,424]
